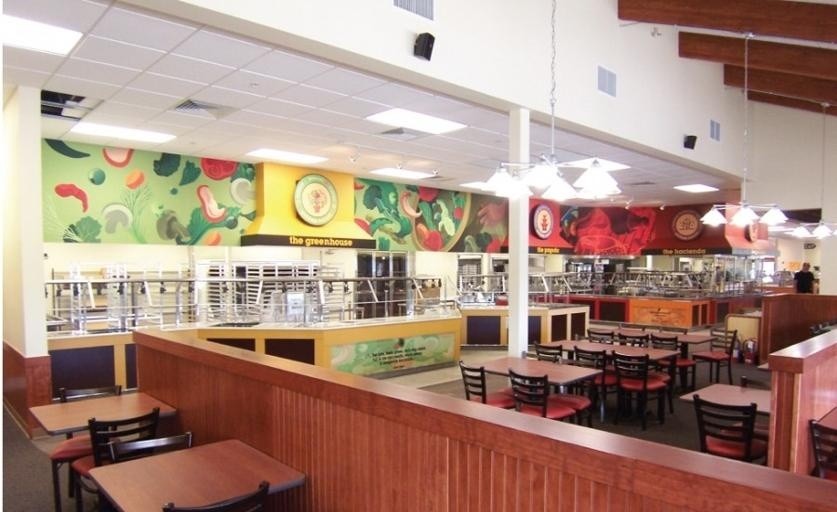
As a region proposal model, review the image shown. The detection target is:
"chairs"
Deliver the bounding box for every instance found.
[51,387,268,512]
[810,419,836,482]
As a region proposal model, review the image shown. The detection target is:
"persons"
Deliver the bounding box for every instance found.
[793,261,813,294]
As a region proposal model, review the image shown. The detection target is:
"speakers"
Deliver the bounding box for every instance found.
[685,135,697,149]
[415,33,435,61]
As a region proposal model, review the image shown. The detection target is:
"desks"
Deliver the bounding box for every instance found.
[26,392,305,512]
